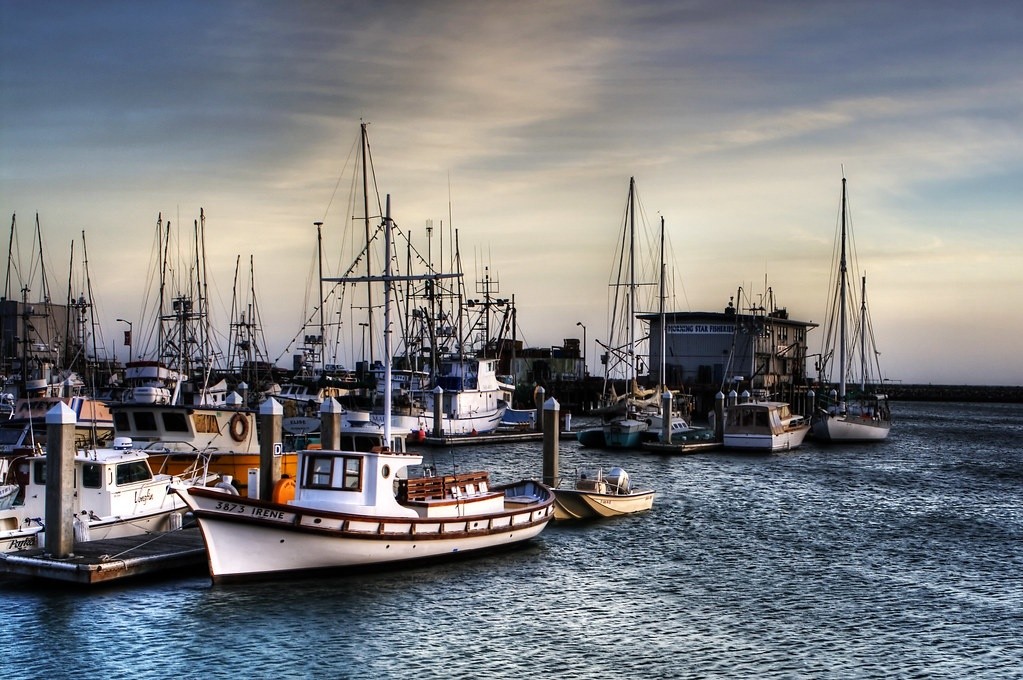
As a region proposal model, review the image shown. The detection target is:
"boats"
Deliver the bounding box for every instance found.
[722,401,813,455]
[163,193,558,587]
[547,466,657,522]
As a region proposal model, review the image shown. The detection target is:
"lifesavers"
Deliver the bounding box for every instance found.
[229,414,249,441]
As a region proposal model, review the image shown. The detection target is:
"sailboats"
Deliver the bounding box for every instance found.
[1,120,892,555]
